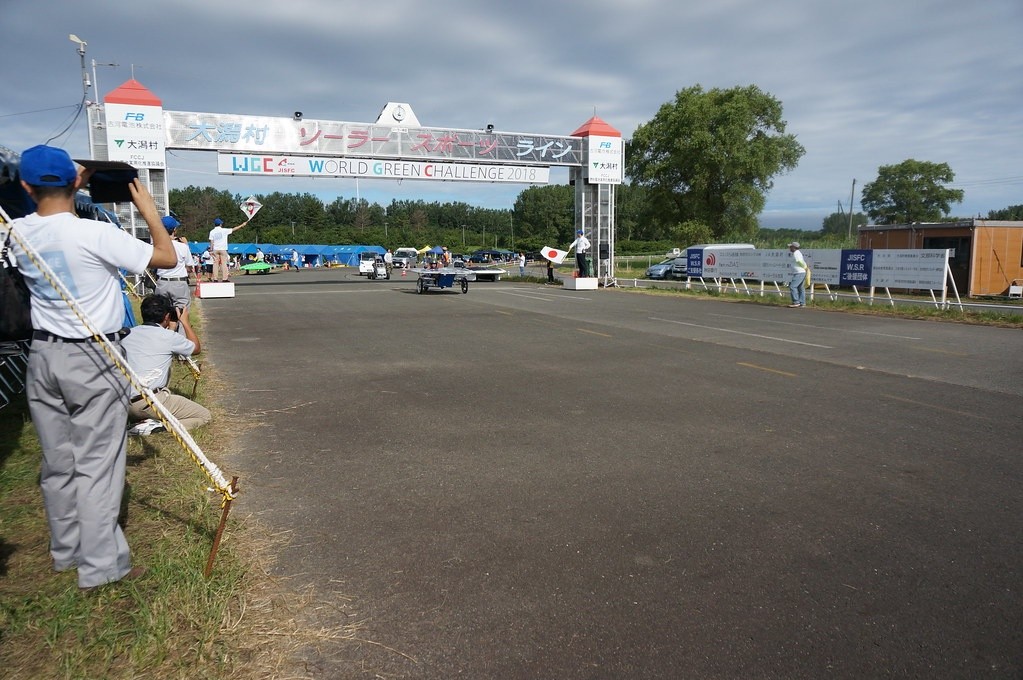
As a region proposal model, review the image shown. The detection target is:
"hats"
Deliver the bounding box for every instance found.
[577,230,583,234]
[161,216,180,230]
[19,145,77,186]
[788,242,800,248]
[207,246,210,249]
[215,219,223,225]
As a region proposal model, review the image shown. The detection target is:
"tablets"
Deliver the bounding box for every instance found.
[88,169,140,204]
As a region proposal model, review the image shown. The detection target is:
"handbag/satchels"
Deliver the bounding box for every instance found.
[804,268,811,289]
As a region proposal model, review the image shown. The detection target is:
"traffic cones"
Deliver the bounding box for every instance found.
[401,269,407,277]
[307,262,311,270]
[284,262,287,270]
[194,273,201,297]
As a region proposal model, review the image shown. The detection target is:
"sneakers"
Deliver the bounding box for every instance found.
[127,419,168,437]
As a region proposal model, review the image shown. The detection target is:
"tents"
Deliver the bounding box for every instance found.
[417,245,444,262]
[185,242,389,271]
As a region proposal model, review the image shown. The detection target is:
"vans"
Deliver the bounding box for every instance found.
[393,248,418,270]
[672,245,754,279]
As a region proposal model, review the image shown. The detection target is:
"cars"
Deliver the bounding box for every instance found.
[647,259,672,279]
[453,251,535,268]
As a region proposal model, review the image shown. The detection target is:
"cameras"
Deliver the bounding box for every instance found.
[170,306,183,322]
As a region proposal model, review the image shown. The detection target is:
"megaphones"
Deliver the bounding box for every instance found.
[487,125,494,131]
[295,112,302,119]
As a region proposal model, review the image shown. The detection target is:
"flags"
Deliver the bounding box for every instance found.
[240,195,262,219]
[540,246,567,264]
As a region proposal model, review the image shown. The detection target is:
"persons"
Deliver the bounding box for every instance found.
[301,254,305,267]
[0,144,177,590]
[118,293,212,437]
[519,252,525,275]
[193,247,213,280]
[292,249,300,270]
[547,260,555,284]
[786,242,807,307]
[570,229,590,277]
[153,216,195,363]
[209,219,247,283]
[254,248,264,273]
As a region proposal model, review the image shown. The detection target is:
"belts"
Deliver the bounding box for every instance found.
[33,329,121,343]
[158,278,187,281]
[129,388,162,405]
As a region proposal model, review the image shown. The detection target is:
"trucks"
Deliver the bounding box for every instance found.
[666,248,679,258]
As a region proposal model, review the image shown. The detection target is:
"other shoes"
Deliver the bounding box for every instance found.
[787,303,800,308]
[799,304,806,307]
[223,280,230,282]
[80,567,149,596]
[214,280,218,282]
